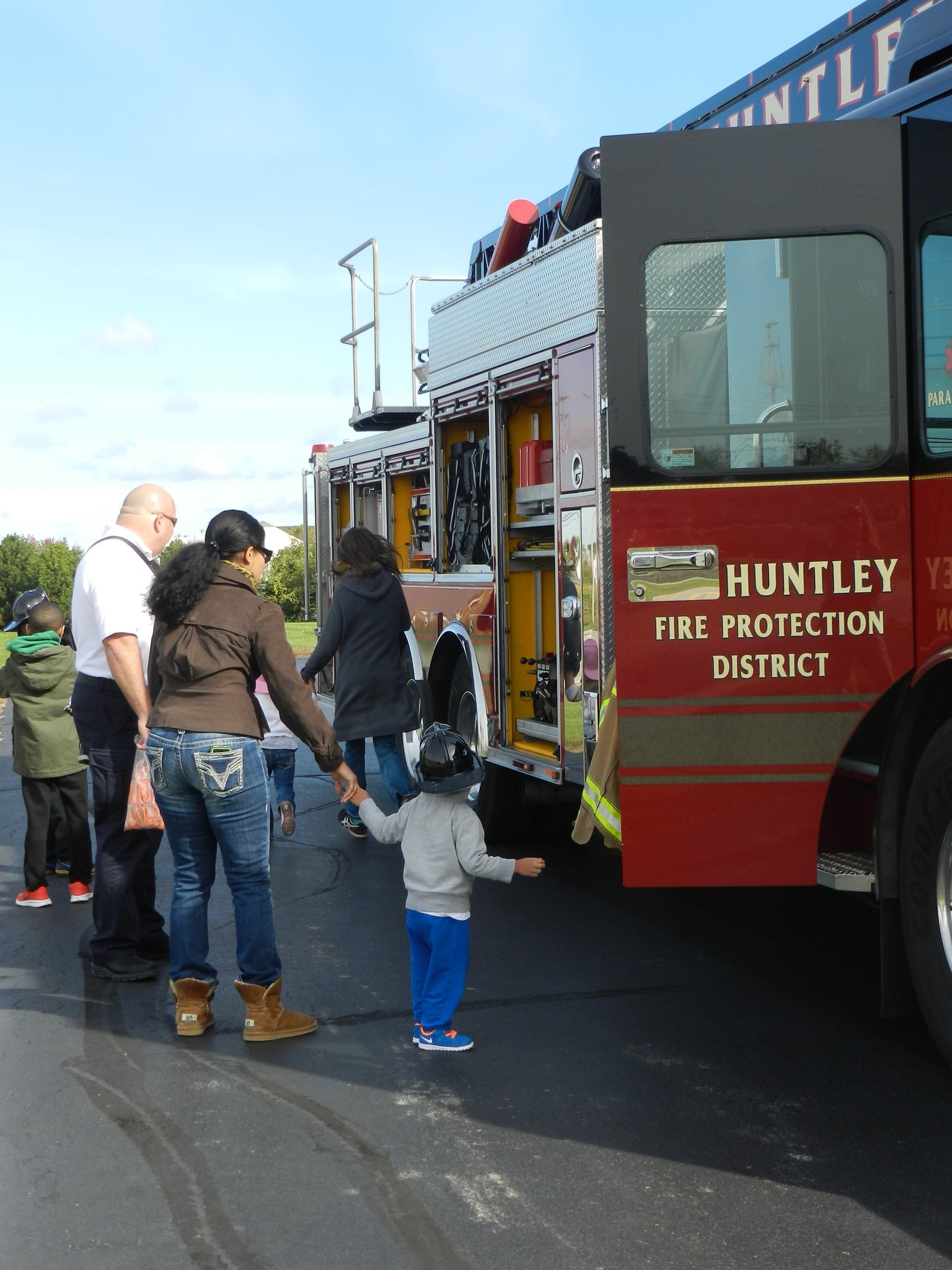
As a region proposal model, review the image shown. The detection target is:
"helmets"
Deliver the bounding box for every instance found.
[415,723,485,794]
[4,586,51,632]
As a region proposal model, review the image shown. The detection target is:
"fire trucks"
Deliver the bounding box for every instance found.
[302,0,952,1074]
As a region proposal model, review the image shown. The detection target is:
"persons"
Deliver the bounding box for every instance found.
[299,527,421,839]
[348,722,545,1050]
[253,673,298,840]
[145,509,359,1044]
[0,587,92,907]
[70,483,177,983]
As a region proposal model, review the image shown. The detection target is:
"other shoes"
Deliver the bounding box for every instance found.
[280,801,295,837]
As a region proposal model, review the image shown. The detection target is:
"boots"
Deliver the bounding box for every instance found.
[169,977,215,1036]
[233,975,317,1041]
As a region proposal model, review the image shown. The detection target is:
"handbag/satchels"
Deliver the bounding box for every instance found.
[124,735,166,830]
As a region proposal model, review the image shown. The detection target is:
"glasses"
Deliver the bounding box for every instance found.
[151,512,177,527]
[255,545,273,563]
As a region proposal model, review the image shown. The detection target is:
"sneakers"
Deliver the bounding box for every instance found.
[142,934,170,958]
[68,881,94,903]
[91,952,159,981]
[412,1023,422,1043]
[337,809,368,837]
[55,858,95,876]
[419,1026,474,1051]
[15,886,53,907]
